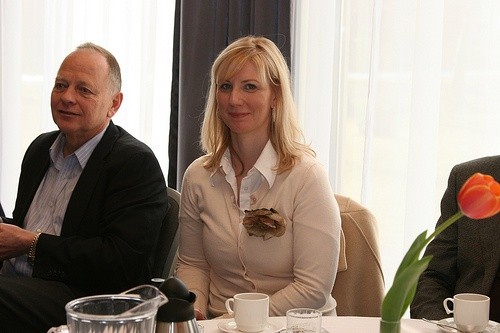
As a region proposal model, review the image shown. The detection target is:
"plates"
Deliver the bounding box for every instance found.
[218,318,275,333]
[277,326,328,333]
[437,318,500,333]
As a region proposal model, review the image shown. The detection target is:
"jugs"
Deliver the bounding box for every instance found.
[64,283,168,333]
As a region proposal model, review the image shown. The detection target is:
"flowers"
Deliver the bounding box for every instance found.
[379,171,500,332]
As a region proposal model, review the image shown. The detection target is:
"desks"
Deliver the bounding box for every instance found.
[147,314,500,333]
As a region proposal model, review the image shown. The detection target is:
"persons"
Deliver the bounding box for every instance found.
[0,42,168,333]
[409,155,500,322]
[175,35,342,321]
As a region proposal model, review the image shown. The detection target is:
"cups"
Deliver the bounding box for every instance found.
[443,294,491,333]
[286,308,322,333]
[225,292,270,332]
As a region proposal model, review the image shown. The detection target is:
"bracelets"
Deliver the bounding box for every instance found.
[26,232,41,265]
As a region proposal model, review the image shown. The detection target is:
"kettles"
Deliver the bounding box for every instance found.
[148,276,198,333]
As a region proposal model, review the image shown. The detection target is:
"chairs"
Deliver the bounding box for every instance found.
[155,186,185,289]
